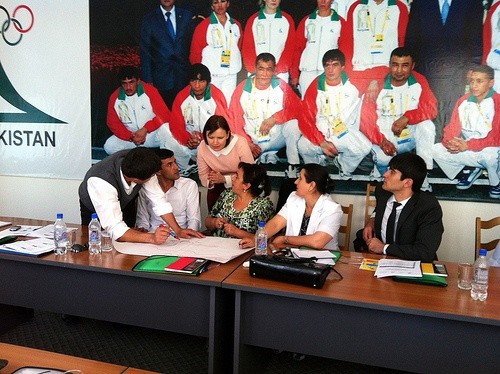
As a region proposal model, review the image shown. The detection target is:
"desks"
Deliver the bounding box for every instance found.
[0,215,500,374]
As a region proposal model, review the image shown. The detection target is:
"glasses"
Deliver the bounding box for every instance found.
[71,241,89,252]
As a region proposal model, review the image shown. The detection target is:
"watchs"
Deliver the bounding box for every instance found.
[283,236,288,245]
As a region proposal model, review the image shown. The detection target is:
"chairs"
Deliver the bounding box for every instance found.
[365,183,378,227]
[475,215,500,264]
[337,204,354,251]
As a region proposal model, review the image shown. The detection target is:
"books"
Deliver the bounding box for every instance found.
[164,257,207,274]
[374,258,448,280]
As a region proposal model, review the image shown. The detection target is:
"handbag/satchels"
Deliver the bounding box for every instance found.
[249,246,344,288]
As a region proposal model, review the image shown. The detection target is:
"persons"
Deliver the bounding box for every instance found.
[488,239,500,267]
[432,65,500,199]
[293,0,347,100]
[62,147,205,322]
[239,163,343,361]
[228,53,302,172]
[197,115,254,214]
[361,152,444,261]
[405,0,500,142]
[205,161,274,238]
[359,47,438,192]
[297,49,388,180]
[139,0,243,111]
[103,64,228,176]
[240,0,295,163]
[133,148,202,234]
[344,0,409,71]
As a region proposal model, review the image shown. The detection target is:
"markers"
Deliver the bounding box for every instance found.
[159,224,180,240]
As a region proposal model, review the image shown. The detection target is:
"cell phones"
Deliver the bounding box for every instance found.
[10,226,21,231]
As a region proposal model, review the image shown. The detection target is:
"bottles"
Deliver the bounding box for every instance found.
[53,213,67,255]
[470,249,490,301]
[88,214,101,255]
[255,222,267,255]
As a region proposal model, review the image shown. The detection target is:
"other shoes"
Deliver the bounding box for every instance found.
[419,176,431,193]
[179,162,197,177]
[333,155,352,182]
[61,314,73,323]
[284,163,297,181]
[456,165,483,189]
[487,182,500,198]
[272,348,305,360]
[368,163,382,183]
[260,153,281,165]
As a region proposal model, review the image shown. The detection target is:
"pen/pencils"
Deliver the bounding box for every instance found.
[202,264,221,272]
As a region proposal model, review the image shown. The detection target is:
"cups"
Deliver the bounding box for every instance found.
[101,231,113,252]
[458,263,473,290]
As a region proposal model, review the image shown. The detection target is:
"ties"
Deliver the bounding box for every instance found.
[386,201,401,244]
[165,12,175,41]
[440,0,450,26]
